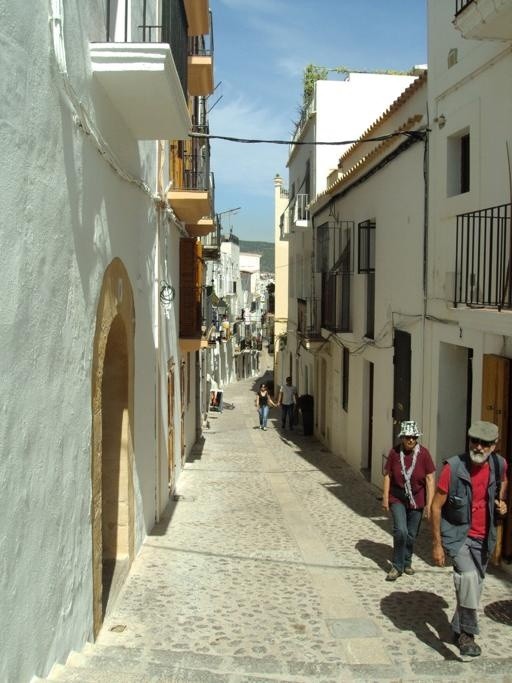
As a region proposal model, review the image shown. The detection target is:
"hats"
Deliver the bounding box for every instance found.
[397,420,424,439]
[467,420,500,443]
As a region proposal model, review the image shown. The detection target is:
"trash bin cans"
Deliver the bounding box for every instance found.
[298,394,314,437]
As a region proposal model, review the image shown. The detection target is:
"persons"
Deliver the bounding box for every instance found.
[277,376,299,430]
[256,383,277,430]
[430,421,507,659]
[381,420,435,581]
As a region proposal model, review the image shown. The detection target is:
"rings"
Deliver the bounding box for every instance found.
[502,509,504,512]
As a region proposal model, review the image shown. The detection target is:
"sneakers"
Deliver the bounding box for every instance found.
[259,425,294,431]
[451,630,481,657]
[385,562,416,581]
